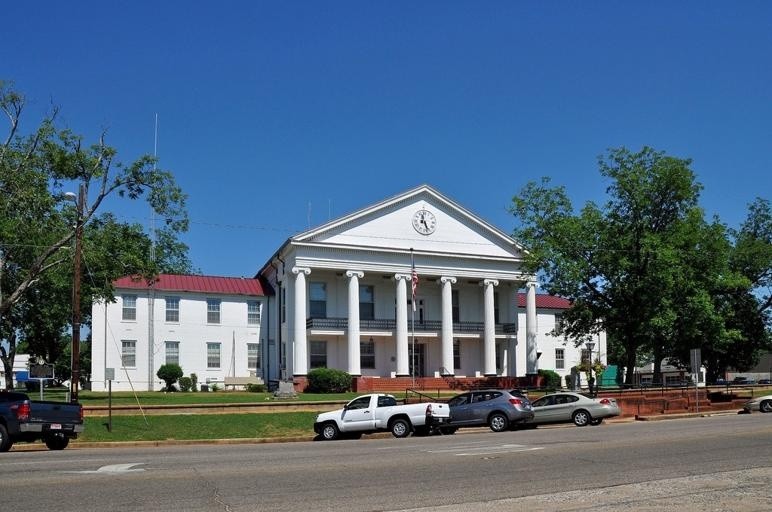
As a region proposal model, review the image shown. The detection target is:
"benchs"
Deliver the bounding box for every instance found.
[224,375,266,391]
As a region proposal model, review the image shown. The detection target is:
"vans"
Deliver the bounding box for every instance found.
[413,388,535,435]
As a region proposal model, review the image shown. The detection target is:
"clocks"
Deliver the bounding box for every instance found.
[410,206,439,236]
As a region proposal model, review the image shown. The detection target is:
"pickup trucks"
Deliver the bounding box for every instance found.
[1,391,84,452]
[314,393,451,440]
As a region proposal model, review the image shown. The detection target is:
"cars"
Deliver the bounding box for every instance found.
[51,379,66,387]
[744,394,772,413]
[712,376,771,384]
[508,392,621,431]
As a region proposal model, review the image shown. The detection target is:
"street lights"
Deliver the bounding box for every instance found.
[61,182,88,403]
[585,335,596,395]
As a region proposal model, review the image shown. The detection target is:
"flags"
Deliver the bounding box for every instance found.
[412,258,419,298]
